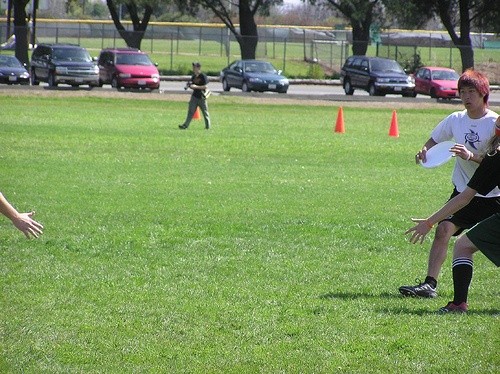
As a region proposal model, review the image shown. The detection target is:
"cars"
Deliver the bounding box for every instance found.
[0,54,31,86]
[98,48,160,92]
[409,66,461,101]
[220,59,289,94]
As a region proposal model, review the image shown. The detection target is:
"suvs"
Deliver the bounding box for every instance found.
[30,42,101,90]
[340,55,416,98]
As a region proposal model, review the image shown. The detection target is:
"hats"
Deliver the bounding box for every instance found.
[192,62,201,68]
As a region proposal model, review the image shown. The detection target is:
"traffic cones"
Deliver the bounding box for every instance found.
[388,109,400,137]
[191,106,201,119]
[334,105,345,134]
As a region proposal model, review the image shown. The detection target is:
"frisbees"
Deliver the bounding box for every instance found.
[421,141,456,169]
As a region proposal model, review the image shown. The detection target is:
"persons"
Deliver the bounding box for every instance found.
[178,63,210,129]
[399,70,500,298]
[404,115,500,315]
[0,191,44,239]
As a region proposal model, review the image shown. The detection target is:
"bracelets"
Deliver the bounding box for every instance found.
[425,218,434,229]
[465,151,473,161]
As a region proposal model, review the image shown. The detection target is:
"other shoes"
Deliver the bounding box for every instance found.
[178,124,185,129]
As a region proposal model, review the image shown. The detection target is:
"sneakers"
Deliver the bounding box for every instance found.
[436,301,468,314]
[399,278,437,299]
